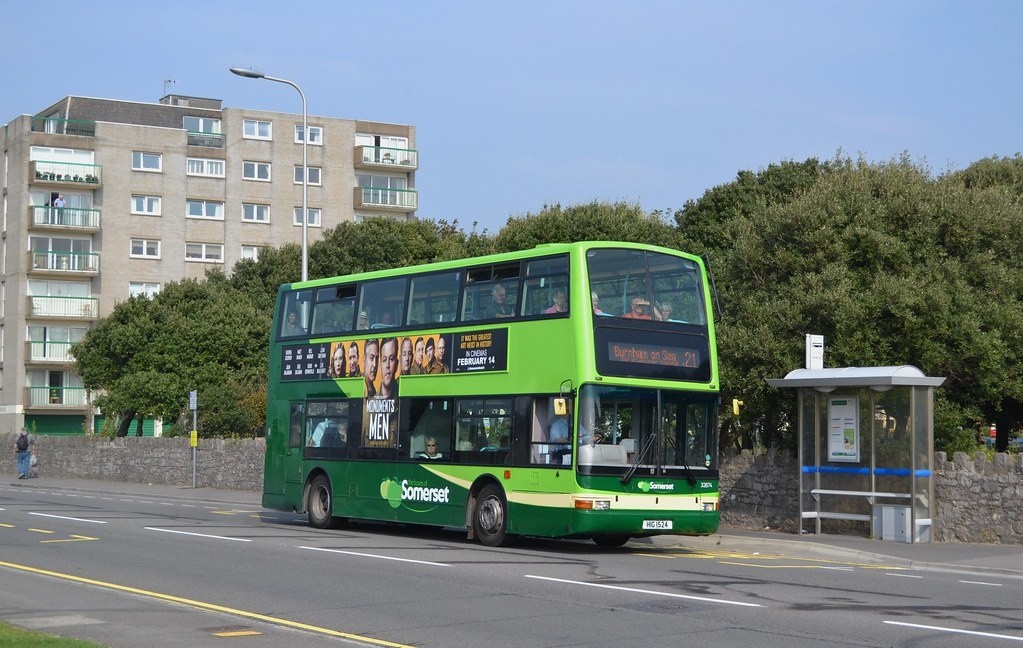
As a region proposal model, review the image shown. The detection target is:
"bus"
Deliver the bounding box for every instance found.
[260,237,744,548]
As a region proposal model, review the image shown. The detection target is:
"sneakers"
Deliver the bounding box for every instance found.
[18,473,25,479]
[23,476,29,479]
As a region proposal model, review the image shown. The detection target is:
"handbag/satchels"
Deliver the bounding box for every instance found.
[30,452,37,467]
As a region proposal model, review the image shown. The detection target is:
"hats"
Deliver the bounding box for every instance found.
[360,311,368,318]
[424,338,434,355]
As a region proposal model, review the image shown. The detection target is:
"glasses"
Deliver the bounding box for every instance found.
[427,444,435,448]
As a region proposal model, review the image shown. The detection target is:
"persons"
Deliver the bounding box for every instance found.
[658,302,673,322]
[363,339,399,448]
[396,338,414,383]
[591,291,604,314]
[14,427,35,479]
[347,341,362,377]
[284,309,306,336]
[412,338,449,375]
[331,342,347,378]
[624,298,662,320]
[545,290,568,314]
[359,312,370,330]
[459,431,474,451]
[485,285,516,318]
[309,408,348,448]
[548,408,601,465]
[418,435,446,462]
[381,312,394,325]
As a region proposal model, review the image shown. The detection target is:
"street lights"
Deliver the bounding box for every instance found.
[230,67,308,330]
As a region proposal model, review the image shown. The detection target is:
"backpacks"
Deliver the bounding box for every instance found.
[17,433,30,452]
